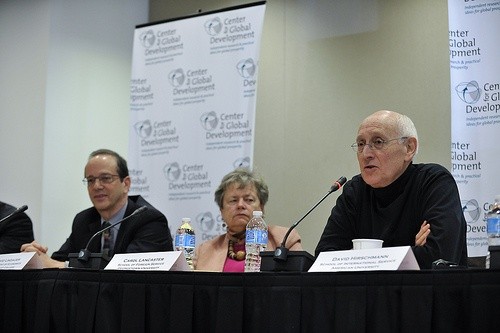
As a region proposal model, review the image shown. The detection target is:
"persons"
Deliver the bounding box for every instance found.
[312,110,468,268]
[188,167,303,271]
[22,149,173,269]
[0,202,34,254]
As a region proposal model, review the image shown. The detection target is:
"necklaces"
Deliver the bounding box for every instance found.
[229,240,246,260]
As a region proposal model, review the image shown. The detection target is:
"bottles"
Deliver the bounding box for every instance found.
[243,211,269,272]
[484,195,500,269]
[174,218,196,270]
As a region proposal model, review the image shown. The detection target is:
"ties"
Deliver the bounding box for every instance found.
[101,221,114,260]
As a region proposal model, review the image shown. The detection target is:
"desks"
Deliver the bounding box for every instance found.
[0,267,500,333]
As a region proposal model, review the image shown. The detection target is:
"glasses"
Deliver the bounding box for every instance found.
[82,173,123,187]
[351,135,409,154]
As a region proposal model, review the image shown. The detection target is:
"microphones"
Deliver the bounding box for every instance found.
[258,176,348,272]
[0,205,28,223]
[68,206,147,270]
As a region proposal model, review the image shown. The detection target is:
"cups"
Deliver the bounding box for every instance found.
[351,238,384,250]
